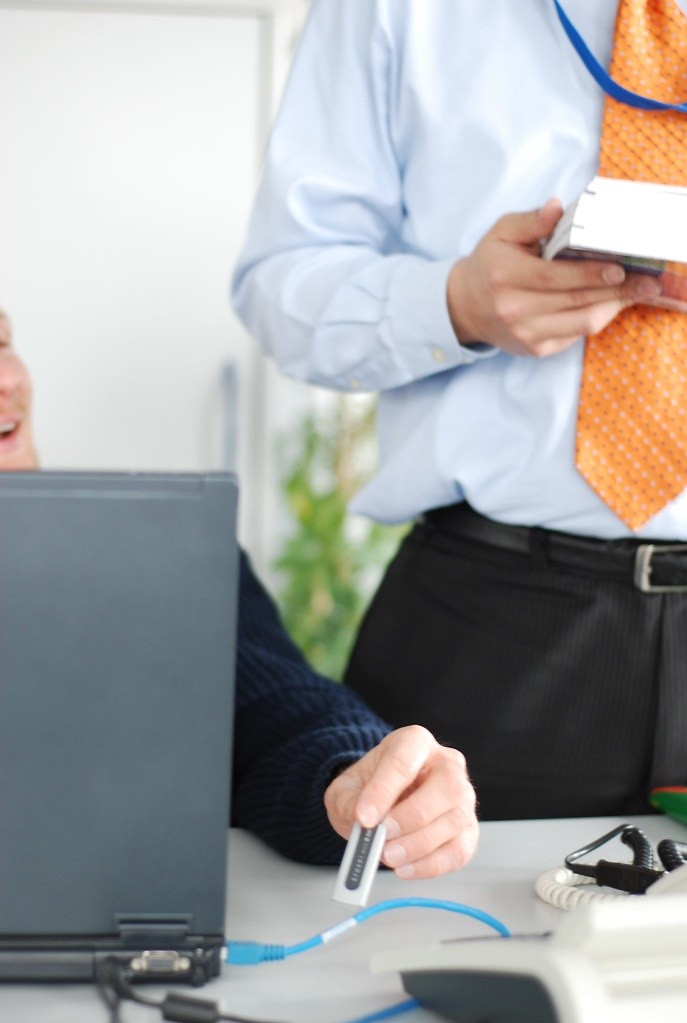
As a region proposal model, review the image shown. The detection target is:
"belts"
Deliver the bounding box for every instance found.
[426,507,687,593]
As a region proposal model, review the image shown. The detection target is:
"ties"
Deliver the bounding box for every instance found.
[571,0,686,534]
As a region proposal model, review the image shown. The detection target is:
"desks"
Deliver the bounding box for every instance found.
[0,814,687,1023]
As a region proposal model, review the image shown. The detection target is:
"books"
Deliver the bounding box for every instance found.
[540,173,687,311]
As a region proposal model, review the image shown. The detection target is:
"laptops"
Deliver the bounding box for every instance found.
[1,469,241,981]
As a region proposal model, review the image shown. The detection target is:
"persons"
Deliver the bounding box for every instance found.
[228,0,687,822]
[0,304,481,880]
